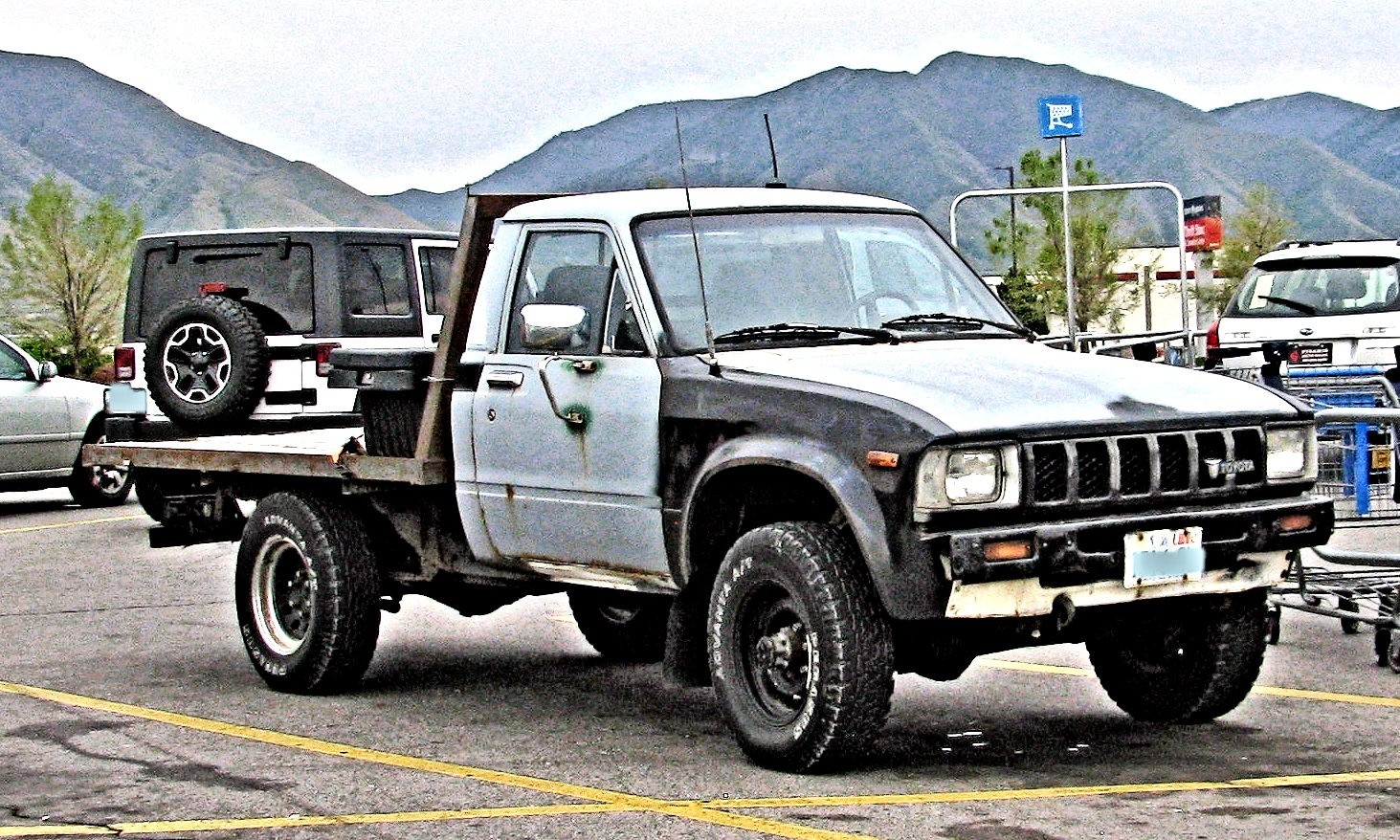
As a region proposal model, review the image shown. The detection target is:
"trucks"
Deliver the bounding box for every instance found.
[637,228,968,345]
[78,173,1339,779]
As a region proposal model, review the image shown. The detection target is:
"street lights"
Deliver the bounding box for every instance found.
[992,165,1017,277]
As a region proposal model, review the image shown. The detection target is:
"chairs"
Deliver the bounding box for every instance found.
[1288,276,1366,312]
[546,266,628,347]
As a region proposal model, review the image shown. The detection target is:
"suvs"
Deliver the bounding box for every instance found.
[1207,235,1400,485]
[102,223,540,533]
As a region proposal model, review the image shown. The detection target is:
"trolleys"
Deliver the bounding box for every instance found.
[1197,337,1400,675]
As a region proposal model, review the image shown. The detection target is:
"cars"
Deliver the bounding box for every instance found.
[0,332,135,509]
